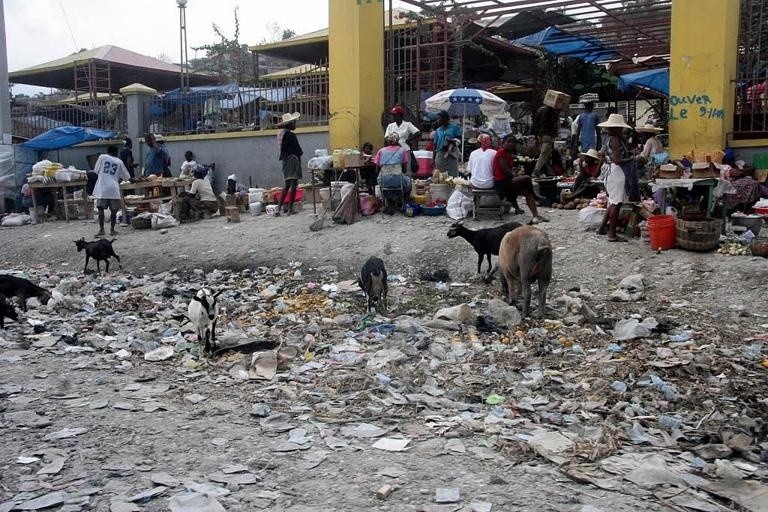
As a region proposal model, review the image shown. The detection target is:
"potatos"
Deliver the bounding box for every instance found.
[552,198,588,209]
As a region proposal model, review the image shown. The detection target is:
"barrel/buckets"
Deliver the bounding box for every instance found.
[430,183,450,203]
[647,215,676,251]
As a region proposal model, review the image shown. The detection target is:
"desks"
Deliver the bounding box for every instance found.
[656,176,728,235]
[30,180,87,224]
[119,177,195,224]
[311,164,374,215]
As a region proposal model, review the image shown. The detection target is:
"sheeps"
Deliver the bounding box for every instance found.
[349,255,391,314]
[72,236,120,274]
[446,219,553,322]
[187,286,226,358]
[0,274,53,332]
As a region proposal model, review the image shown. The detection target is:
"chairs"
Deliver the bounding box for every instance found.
[380,164,404,214]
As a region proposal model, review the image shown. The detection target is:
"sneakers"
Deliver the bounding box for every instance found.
[516,209,524,214]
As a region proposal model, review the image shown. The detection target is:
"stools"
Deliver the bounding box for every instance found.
[225,206,240,223]
[472,188,506,220]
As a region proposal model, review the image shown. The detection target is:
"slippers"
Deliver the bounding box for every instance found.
[275,213,280,216]
[288,212,296,216]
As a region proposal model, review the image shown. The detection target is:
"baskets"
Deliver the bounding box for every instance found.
[129,216,151,231]
[676,218,724,251]
[751,238,764,255]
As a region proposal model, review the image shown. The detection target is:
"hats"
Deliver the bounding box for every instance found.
[388,105,404,114]
[596,113,633,130]
[635,124,664,133]
[274,111,301,127]
[228,173,236,181]
[154,134,167,142]
[437,110,448,116]
[578,149,600,160]
[387,132,399,144]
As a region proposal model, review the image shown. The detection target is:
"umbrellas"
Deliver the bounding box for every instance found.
[617,66,669,96]
[426,86,507,163]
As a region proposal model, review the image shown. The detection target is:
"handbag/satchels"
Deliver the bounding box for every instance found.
[368,146,400,186]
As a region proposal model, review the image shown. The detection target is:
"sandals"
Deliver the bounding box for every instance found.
[94,233,105,238]
[608,235,628,242]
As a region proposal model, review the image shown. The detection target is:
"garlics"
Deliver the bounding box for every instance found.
[717,239,750,257]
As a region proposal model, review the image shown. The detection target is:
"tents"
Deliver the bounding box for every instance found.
[18,125,121,171]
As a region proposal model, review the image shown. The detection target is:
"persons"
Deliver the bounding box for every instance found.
[155,134,173,177]
[570,114,580,147]
[597,113,633,244]
[574,101,602,153]
[119,137,139,196]
[359,142,375,195]
[384,106,420,178]
[178,150,196,194]
[634,124,664,164]
[91,145,131,238]
[189,165,218,219]
[467,134,497,190]
[530,106,559,180]
[274,112,305,217]
[374,132,411,208]
[430,110,462,178]
[142,132,164,176]
[561,148,602,202]
[492,134,550,223]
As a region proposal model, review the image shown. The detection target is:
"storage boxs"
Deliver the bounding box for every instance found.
[543,89,570,109]
[659,164,682,178]
[276,190,303,203]
[692,162,719,177]
[413,149,433,176]
[754,169,768,187]
